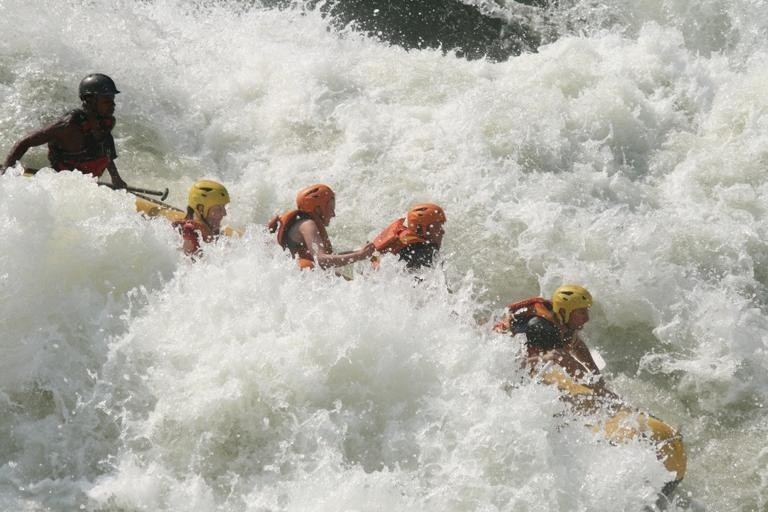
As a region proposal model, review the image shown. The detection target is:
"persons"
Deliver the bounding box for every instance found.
[2,72,127,189]
[492,284,620,411]
[267,184,376,281]
[171,180,230,265]
[364,203,454,294]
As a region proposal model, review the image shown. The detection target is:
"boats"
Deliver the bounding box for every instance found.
[12,167,685,499]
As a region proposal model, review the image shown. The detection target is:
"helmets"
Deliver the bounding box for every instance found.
[551,284,593,324]
[78,73,121,99]
[187,180,446,239]
